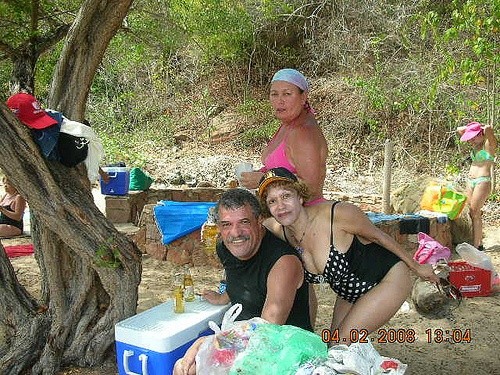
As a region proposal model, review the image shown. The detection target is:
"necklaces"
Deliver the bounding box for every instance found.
[286,209,309,254]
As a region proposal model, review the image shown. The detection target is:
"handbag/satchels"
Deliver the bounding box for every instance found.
[455,242,493,271]
[196,303,407,375]
[414,232,451,265]
[60,119,91,166]
[420,184,467,221]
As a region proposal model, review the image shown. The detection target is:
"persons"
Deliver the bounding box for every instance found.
[257,167,440,352]
[239,68,328,332]
[172,188,315,375]
[457,122,496,251]
[0,175,27,237]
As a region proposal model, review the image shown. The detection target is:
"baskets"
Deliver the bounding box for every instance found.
[447,262,491,297]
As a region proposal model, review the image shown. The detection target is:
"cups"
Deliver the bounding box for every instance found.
[235,163,253,187]
[229,180,237,188]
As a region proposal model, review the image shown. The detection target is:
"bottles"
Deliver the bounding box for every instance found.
[184,269,195,302]
[172,275,185,313]
[201,210,220,255]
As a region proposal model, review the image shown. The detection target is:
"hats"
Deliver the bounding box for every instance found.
[7,93,58,129]
[259,167,298,199]
[30,112,63,157]
[459,122,482,142]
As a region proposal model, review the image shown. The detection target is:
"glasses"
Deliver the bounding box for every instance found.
[436,278,463,313]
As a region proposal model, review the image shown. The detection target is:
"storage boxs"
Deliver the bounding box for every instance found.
[100,167,132,195]
[114,295,231,375]
[448,261,492,298]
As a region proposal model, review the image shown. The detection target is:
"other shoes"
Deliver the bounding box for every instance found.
[477,245,483,251]
